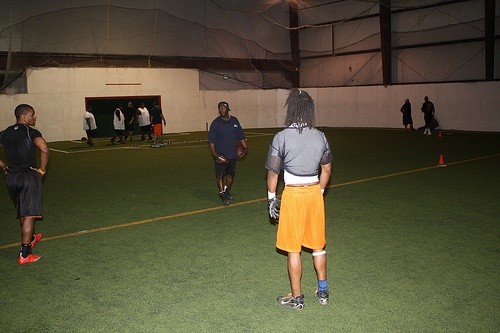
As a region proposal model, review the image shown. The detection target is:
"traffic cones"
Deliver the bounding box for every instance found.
[437,154,447,167]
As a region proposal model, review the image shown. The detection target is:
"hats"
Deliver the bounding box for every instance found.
[218,102,231,111]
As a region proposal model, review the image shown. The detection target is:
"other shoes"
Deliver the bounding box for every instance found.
[219,185,233,205]
[86,141,95,147]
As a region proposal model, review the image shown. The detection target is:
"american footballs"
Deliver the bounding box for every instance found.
[237,146,245,159]
[216,155,230,164]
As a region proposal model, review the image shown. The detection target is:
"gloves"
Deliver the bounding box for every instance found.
[267,198,280,219]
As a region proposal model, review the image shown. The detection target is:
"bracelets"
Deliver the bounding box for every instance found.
[39,168,46,175]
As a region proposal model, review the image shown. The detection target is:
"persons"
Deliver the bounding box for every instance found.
[264,90,332,310]
[422,96,435,125]
[110,104,127,145]
[124,102,135,140]
[136,103,152,142]
[151,102,166,142]
[209,102,248,206]
[400,98,413,133]
[83,106,97,147]
[0,104,49,265]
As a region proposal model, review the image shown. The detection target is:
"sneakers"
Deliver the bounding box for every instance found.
[277,293,304,310]
[314,287,329,305]
[18,252,41,265]
[30,233,42,248]
